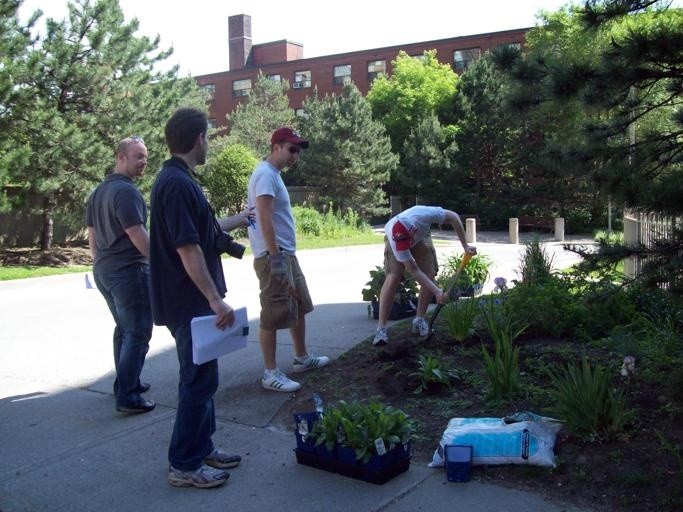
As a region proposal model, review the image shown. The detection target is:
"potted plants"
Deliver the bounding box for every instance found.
[293,400,418,485]
[361,252,494,320]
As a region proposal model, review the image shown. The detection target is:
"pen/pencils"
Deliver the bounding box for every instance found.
[248,218,255,228]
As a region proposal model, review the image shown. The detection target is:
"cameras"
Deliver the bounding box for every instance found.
[216,233,246,259]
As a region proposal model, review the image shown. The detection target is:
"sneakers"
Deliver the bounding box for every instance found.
[168,464,229,488]
[293,354,329,373]
[412,318,436,336]
[372,328,388,345]
[204,449,241,468]
[262,369,301,392]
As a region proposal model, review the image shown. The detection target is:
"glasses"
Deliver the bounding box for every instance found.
[289,146,300,153]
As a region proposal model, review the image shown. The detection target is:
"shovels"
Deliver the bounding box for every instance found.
[420,253,472,345]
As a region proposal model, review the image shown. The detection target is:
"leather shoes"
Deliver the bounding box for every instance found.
[116,400,156,412]
[139,383,150,393]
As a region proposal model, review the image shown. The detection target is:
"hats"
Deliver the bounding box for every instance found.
[272,128,309,149]
[392,219,417,251]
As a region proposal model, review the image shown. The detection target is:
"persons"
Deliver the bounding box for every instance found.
[245,127,329,392]
[146,108,257,489]
[369,202,476,348]
[84,135,156,415]
